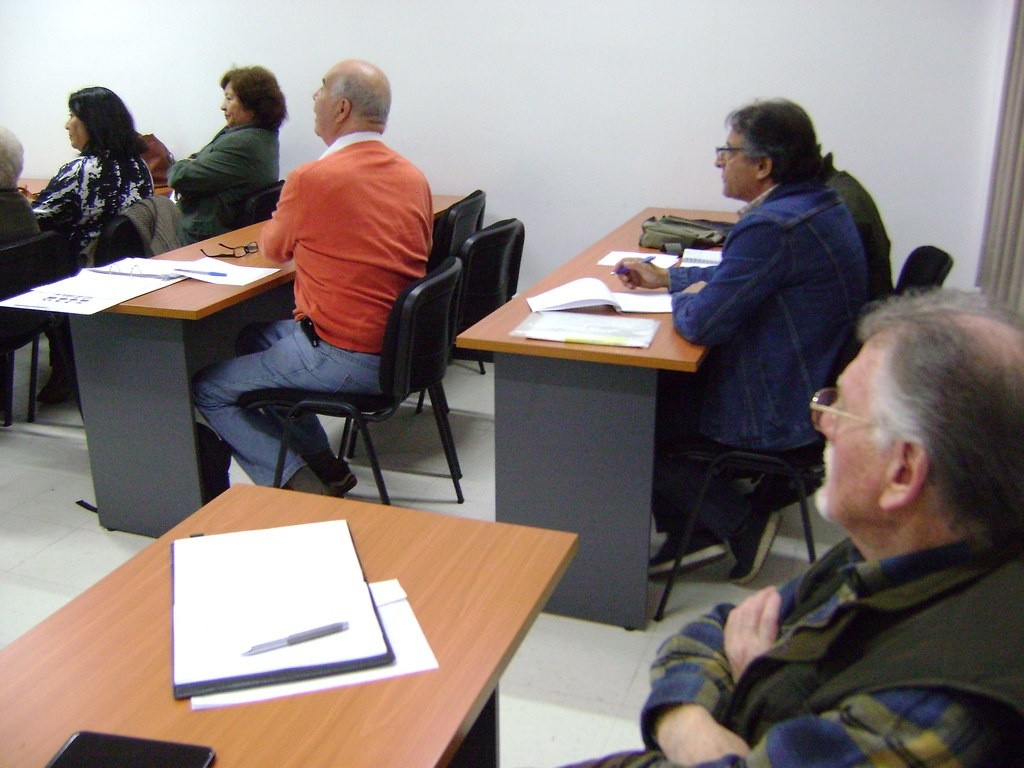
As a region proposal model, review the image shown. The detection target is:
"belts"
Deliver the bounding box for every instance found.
[300,315,319,347]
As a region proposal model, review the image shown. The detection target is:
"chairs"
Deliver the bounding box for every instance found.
[454,219,526,365]
[244,256,464,507]
[95,196,184,265]
[0,230,67,427]
[237,180,285,229]
[656,442,828,622]
[895,245,955,295]
[427,188,490,375]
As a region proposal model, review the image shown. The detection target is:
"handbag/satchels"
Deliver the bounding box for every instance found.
[136,134,177,187]
[638,214,738,251]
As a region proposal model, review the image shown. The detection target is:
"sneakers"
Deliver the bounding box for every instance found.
[648,528,726,576]
[729,507,782,584]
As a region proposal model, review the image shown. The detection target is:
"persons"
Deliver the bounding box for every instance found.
[18,87,154,405]
[0,129,43,318]
[614,99,897,581]
[549,289,1024,768]
[191,58,434,498]
[166,67,286,247]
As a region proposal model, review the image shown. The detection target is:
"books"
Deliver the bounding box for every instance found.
[680,249,722,269]
[171,521,395,700]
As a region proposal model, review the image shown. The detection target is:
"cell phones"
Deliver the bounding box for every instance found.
[44,730,216,768]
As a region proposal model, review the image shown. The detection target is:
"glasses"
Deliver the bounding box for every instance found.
[715,145,759,160]
[809,388,936,485]
[200,241,258,258]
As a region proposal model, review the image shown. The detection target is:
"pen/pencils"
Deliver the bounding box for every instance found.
[240,621,349,656]
[610,257,654,275]
[175,269,226,277]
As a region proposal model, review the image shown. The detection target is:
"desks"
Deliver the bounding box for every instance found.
[0,482,580,768]
[454,206,744,630]
[68,193,466,539]
[21,179,174,200]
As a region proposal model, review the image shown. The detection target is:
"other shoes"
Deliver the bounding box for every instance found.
[36,365,80,403]
[327,460,357,494]
